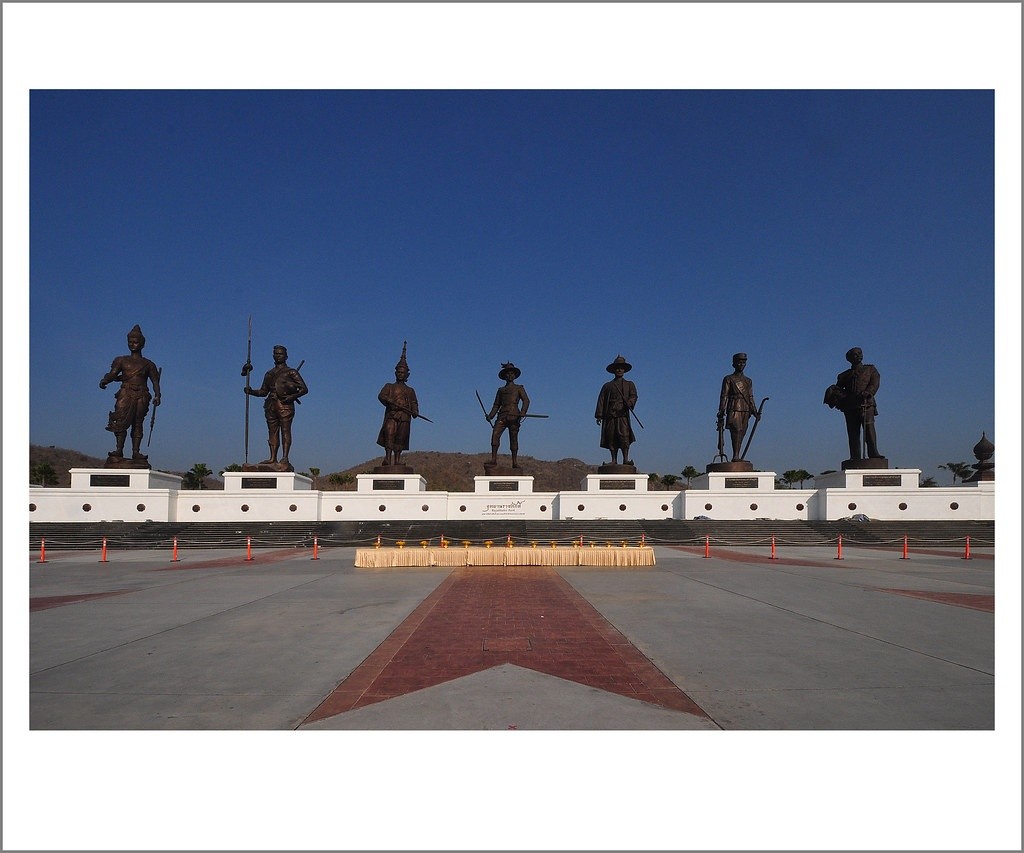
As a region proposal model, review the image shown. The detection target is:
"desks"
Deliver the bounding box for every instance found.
[355,547,655,567]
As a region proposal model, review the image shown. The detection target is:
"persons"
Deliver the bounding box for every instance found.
[836,348,886,459]
[484,360,530,468]
[376,340,419,466]
[99,325,162,459]
[717,353,761,462]
[595,354,638,465]
[244,345,308,464]
